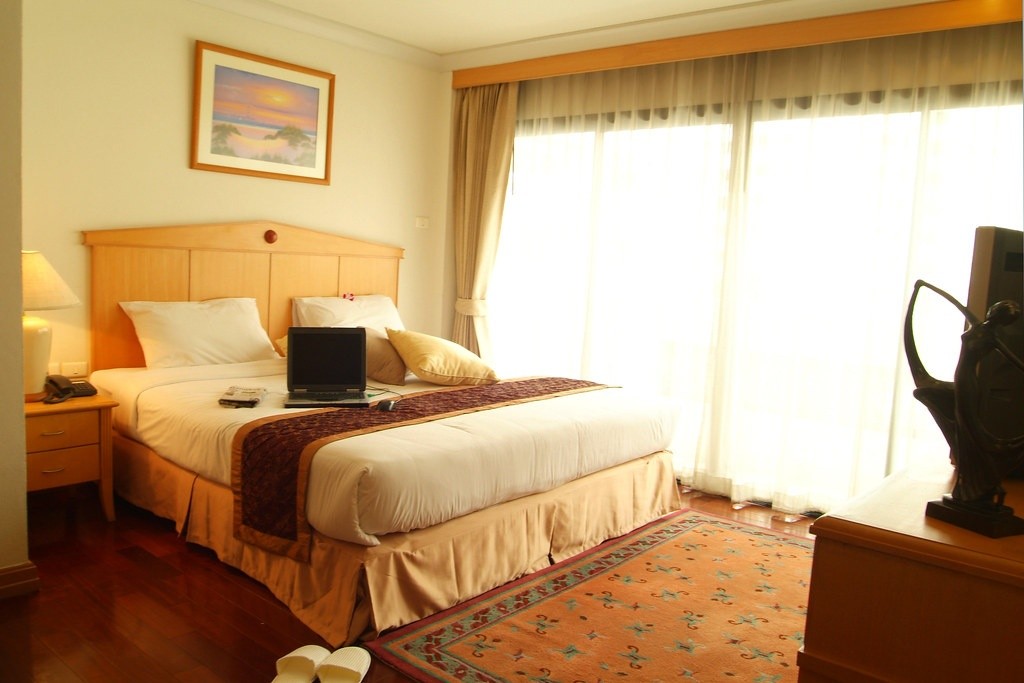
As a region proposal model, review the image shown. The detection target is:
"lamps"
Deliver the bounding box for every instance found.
[22,249,83,395]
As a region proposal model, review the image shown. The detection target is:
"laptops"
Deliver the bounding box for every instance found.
[284,328,370,408]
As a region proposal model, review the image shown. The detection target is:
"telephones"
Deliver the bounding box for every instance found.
[44,374,98,398]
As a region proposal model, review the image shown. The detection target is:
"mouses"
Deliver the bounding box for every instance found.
[377,400,397,412]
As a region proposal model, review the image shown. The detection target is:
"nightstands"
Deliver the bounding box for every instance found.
[24,395,120,521]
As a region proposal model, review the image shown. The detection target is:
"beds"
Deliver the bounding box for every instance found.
[82,216,683,650]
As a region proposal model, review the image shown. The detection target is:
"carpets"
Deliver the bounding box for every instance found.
[362,507,815,682]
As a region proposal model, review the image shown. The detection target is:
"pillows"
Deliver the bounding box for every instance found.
[118,296,502,388]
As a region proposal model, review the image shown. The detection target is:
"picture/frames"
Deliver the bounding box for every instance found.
[188,39,338,187]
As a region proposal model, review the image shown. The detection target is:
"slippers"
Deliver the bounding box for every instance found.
[271,645,331,683]
[317,647,371,683]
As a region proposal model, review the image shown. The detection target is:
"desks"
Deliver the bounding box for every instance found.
[796,462,1024,683]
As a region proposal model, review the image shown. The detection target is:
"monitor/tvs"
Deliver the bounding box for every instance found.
[964,225,1024,482]
